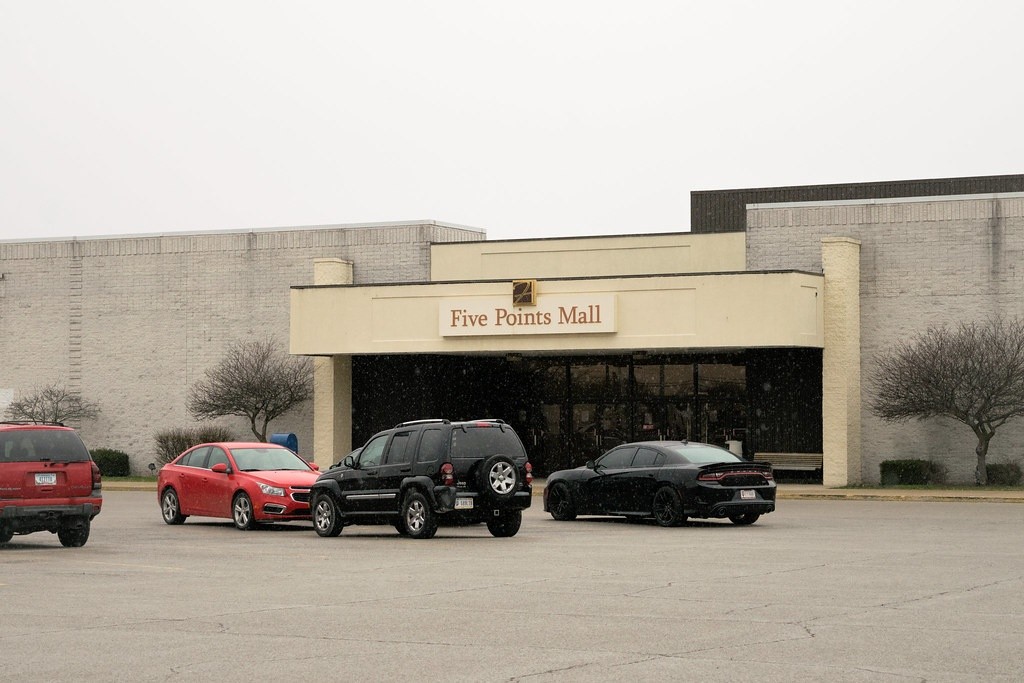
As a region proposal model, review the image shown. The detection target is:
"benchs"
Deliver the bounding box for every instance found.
[753,452,823,484]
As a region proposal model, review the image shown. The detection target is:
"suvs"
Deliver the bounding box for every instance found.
[0,420,103,547]
[310,418,533,539]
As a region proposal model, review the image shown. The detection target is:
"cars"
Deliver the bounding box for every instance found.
[542,438,777,527]
[157,442,324,529]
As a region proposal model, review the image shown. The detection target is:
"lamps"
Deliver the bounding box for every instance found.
[0,273,12,298]
[505,351,522,362]
[633,351,647,359]
